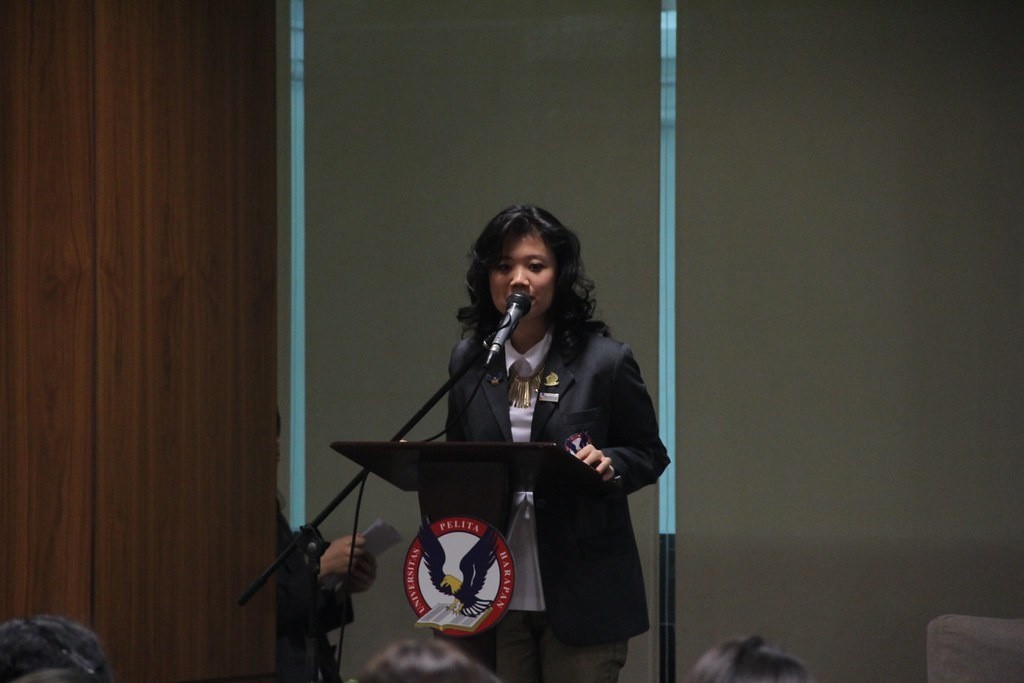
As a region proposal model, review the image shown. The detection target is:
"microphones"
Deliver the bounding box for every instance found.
[486,292,532,369]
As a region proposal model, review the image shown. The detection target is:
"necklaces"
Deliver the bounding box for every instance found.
[509,355,548,410]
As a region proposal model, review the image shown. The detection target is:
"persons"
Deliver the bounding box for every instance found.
[271,406,375,683]
[361,635,505,683]
[688,636,817,683]
[430,203,673,683]
[0,616,119,683]
